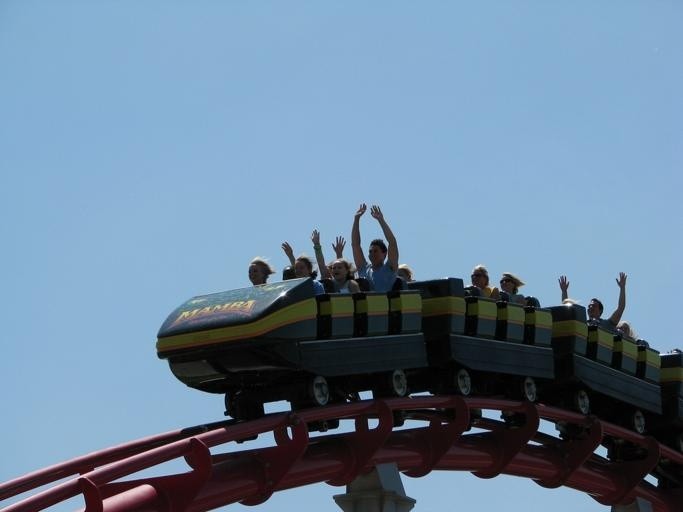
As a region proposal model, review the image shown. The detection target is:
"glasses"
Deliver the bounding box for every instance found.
[500,279,512,284]
[471,274,481,278]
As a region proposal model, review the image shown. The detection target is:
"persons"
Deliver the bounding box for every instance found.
[558,272,630,338]
[248,203,412,293]
[471,264,540,307]
